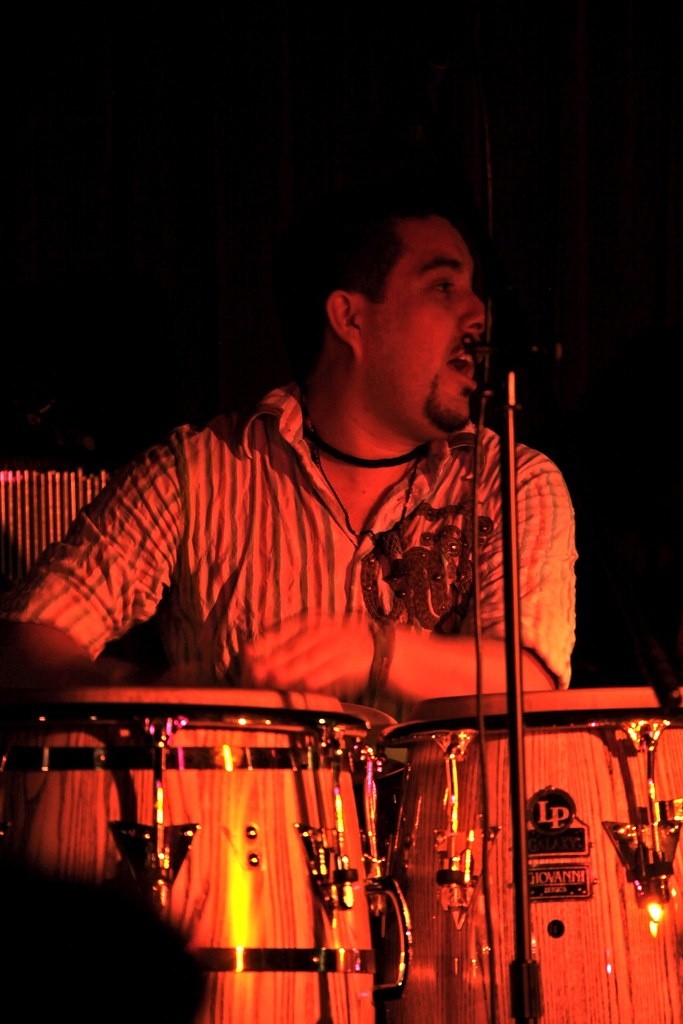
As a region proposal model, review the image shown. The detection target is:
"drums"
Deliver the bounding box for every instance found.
[0,676,373,1024]
[374,687,682,1023]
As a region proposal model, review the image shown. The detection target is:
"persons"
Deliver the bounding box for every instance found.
[0,209,579,806]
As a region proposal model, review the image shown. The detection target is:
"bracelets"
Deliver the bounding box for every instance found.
[370,619,397,692]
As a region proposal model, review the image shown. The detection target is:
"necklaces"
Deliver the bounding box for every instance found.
[302,423,423,467]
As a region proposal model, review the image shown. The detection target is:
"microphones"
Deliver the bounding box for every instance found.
[27,399,55,426]
